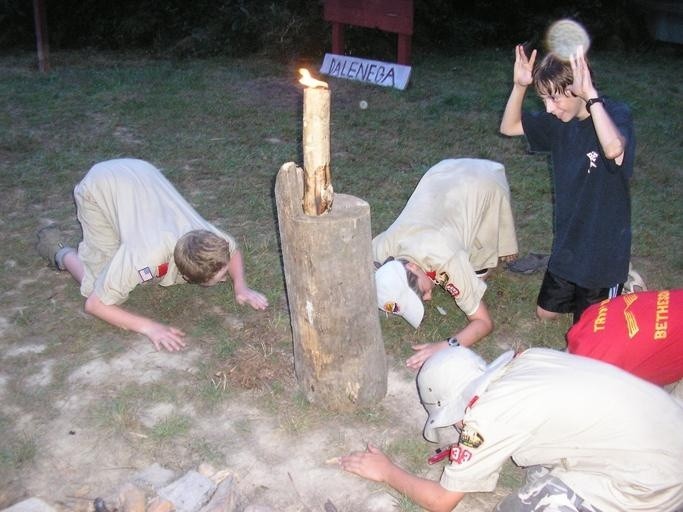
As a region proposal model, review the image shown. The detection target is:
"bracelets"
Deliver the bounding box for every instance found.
[585,95,606,114]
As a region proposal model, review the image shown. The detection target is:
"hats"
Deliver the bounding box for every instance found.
[418,345,515,443]
[375,261,424,329]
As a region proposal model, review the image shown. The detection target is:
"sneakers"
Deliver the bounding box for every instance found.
[621,262,648,294]
[35,224,67,266]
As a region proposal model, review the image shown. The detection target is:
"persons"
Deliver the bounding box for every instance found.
[35,158,268,353]
[339,346,683,512]
[371,157,551,369]
[500,42,648,328]
[561,282,682,404]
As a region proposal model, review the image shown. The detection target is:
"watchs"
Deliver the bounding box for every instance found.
[446,336,461,348]
